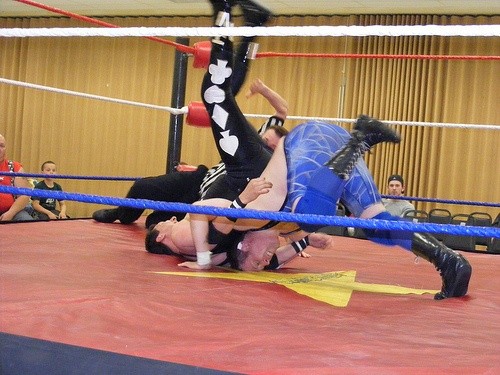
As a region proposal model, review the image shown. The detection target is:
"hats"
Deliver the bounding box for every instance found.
[388,176,403,187]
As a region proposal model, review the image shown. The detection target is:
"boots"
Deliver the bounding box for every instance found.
[235,0,271,66]
[323,115,400,181]
[209,0,240,54]
[411,232,472,299]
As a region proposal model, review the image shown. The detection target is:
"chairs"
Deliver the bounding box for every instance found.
[317,203,500,255]
[25,179,70,221]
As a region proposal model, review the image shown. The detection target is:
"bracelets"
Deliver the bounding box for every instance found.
[291,235,310,254]
[225,196,247,223]
[196,250,212,266]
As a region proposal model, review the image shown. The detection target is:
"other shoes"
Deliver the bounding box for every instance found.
[93,207,119,223]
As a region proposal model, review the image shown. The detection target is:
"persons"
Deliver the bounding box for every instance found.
[379,175,418,219]
[0,132,68,223]
[92,0,472,299]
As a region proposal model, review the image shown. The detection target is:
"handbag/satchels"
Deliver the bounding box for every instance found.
[8,160,38,214]
